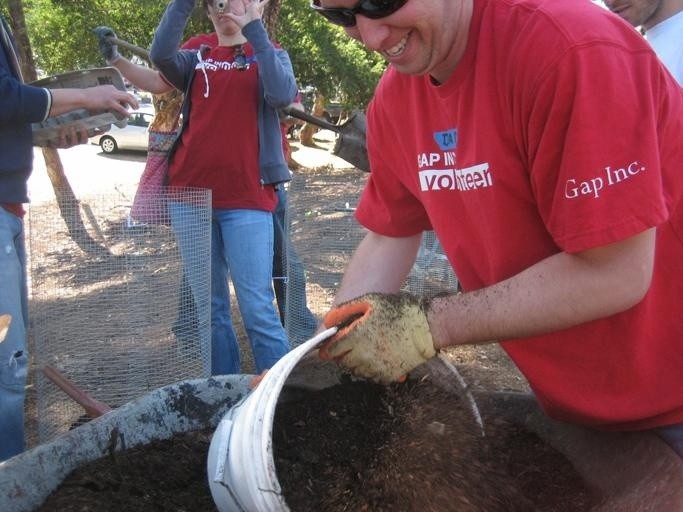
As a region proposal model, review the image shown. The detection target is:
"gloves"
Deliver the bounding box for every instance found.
[320,290,436,385]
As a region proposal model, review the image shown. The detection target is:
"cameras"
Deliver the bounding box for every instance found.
[214,0,229,15]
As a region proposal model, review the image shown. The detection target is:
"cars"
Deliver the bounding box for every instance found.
[91,111,155,154]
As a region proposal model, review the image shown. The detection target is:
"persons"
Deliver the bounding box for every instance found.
[90,22,316,360]
[148,2,299,378]
[0,8,141,461]
[601,0,682,88]
[313,0,682,458]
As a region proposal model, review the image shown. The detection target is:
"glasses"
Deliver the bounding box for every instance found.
[310,0,409,28]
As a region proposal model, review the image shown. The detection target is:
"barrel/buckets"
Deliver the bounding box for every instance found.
[205,324,488,511]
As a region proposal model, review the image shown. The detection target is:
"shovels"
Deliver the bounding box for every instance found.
[106,35,371,172]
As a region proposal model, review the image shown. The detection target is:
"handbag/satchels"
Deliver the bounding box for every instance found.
[128,130,177,225]
